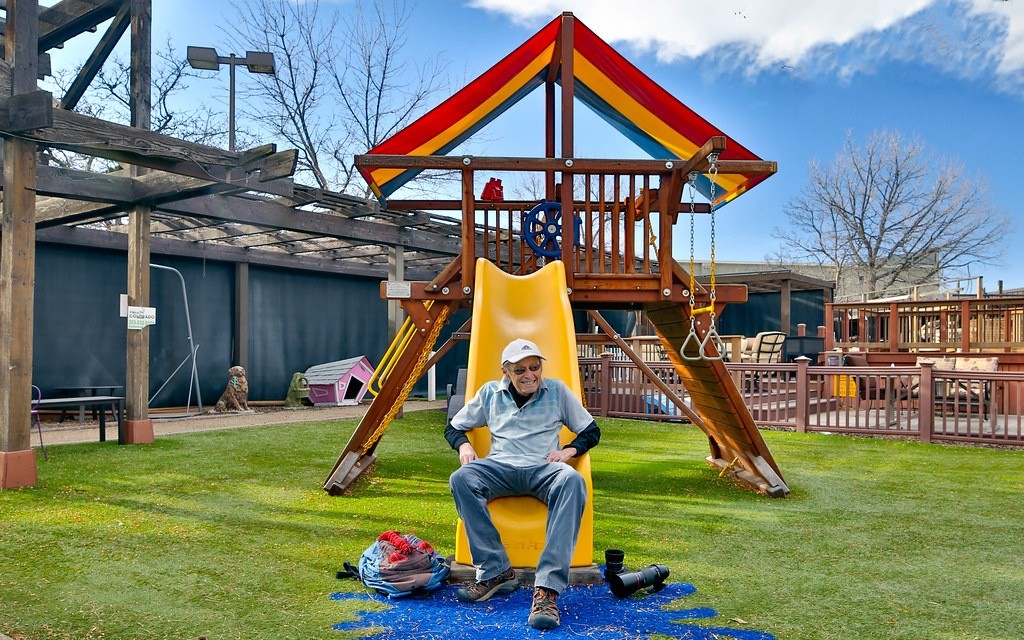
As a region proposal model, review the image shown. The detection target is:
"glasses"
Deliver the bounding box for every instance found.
[508,363,543,376]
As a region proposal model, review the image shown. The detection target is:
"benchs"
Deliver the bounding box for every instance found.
[907,356,1003,420]
[30,393,124,447]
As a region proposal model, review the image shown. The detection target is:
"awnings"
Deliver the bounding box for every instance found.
[356,11,773,213]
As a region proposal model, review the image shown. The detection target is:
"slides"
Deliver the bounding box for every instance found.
[454,258,597,565]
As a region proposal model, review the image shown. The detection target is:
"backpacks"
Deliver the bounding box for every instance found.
[336,528,449,600]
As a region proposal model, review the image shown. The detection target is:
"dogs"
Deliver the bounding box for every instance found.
[214,366,252,412]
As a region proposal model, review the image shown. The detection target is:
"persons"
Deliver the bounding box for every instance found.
[443,338,601,629]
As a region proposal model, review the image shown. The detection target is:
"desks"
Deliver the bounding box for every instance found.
[59,384,123,422]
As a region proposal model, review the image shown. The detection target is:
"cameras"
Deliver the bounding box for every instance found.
[603,550,626,582]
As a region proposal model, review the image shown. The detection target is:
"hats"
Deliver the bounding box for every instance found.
[501,337,548,364]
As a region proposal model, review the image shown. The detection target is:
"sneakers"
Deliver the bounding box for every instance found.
[456,566,520,604]
[527,586,563,629]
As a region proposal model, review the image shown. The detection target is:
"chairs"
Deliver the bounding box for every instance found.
[844,355,916,417]
[578,330,788,394]
